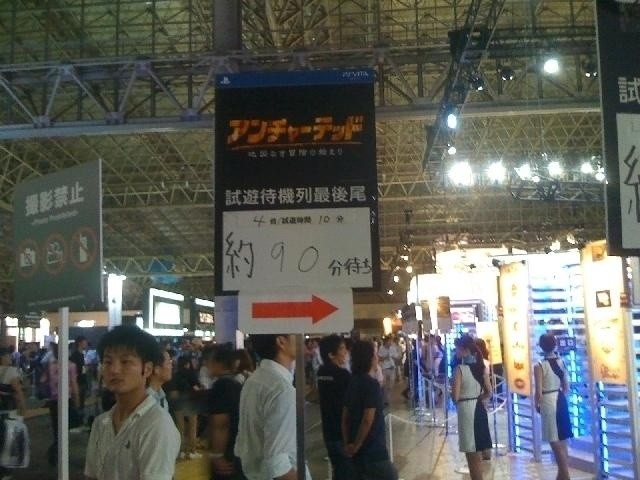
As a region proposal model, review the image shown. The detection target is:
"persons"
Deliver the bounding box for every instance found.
[534,334,573,480]
[148,337,213,459]
[203,341,246,480]
[233,330,312,479]
[452,337,491,480]
[339,339,398,479]
[236,349,255,384]
[83,325,182,480]
[0,336,99,480]
[317,335,351,478]
[371,332,448,406]
[475,339,492,460]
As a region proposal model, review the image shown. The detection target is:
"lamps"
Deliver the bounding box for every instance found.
[579,53,598,79]
[469,74,485,91]
[495,62,515,82]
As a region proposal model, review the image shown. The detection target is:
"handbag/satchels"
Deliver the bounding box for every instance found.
[2,417,31,470]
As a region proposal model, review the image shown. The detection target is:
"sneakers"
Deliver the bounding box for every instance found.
[187,451,202,458]
[178,451,185,458]
